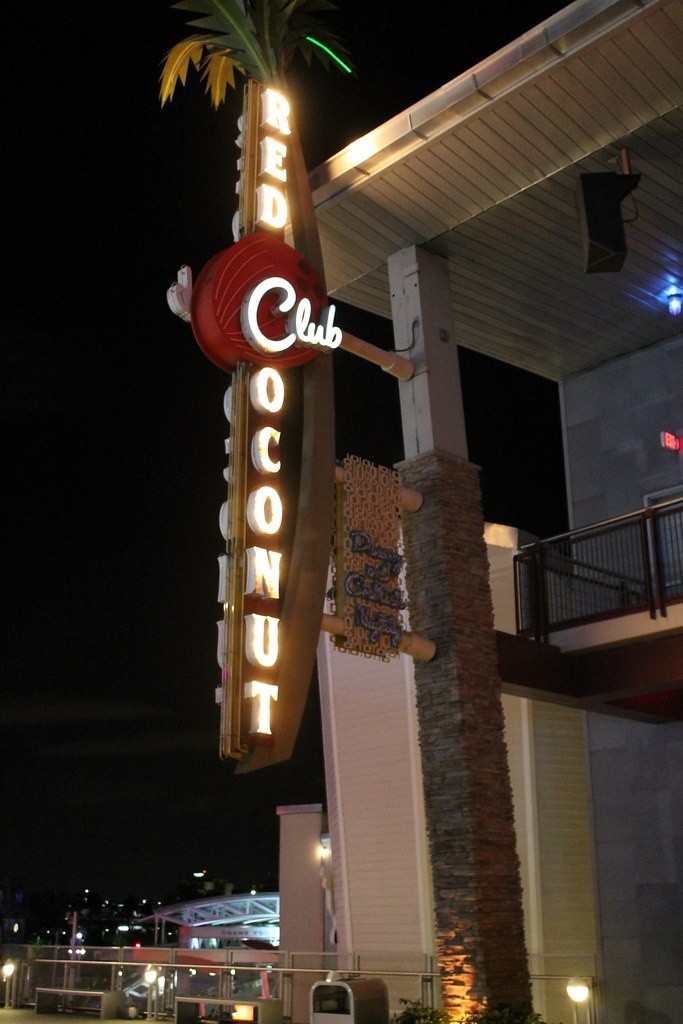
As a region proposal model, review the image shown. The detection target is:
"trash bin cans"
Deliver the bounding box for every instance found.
[309,976,390,1024]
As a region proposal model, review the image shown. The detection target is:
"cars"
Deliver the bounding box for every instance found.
[116,962,280,1021]
